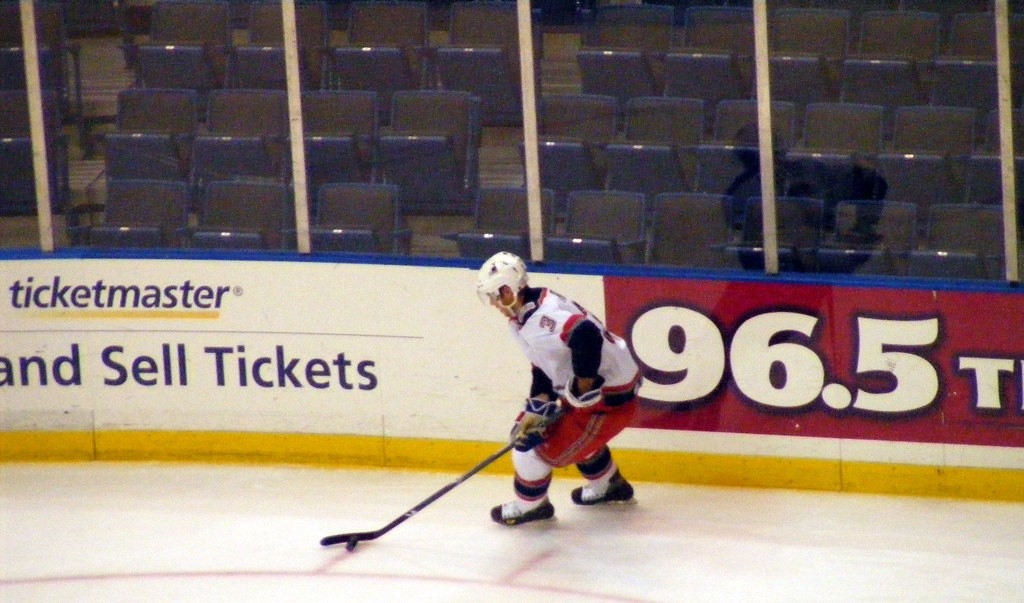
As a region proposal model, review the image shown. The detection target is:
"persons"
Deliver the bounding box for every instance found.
[476,252,644,527]
[722,116,888,274]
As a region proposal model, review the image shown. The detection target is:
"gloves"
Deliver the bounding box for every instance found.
[561,374,605,416]
[510,398,558,452]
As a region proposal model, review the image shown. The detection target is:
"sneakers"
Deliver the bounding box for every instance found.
[571,469,634,505]
[491,496,553,526]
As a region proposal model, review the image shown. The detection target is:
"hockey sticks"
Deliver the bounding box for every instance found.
[319,408,571,547]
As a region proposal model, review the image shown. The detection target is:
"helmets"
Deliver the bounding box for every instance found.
[475,251,528,297]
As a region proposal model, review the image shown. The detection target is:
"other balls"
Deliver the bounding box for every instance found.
[346,534,359,551]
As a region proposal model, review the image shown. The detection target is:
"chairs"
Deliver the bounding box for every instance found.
[0,0,1024,273]
